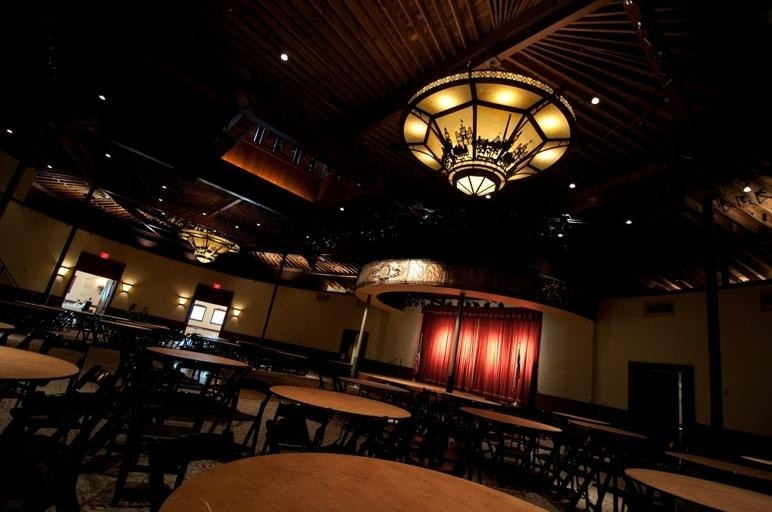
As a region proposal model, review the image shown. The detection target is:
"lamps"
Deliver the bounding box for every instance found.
[57,266,71,278]
[177,295,189,306]
[121,281,133,294]
[177,220,241,267]
[398,59,578,200]
